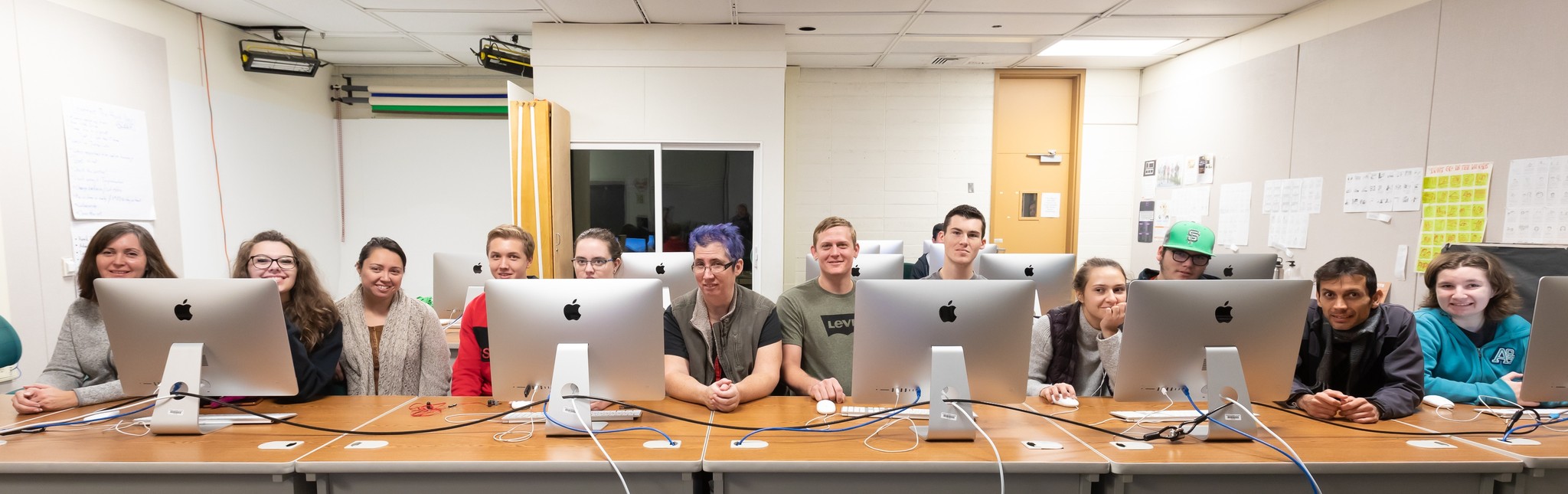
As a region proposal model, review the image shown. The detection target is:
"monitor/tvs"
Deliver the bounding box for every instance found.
[1519,275,1568,402]
[929,243,997,275]
[805,240,904,283]
[613,252,699,314]
[433,252,496,319]
[1203,254,1278,280]
[979,253,1076,317]
[923,240,933,254]
[852,279,1036,439]
[485,278,665,436]
[1114,280,1314,441]
[93,278,299,435]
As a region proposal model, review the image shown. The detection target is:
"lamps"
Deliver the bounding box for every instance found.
[470,35,533,80]
[238,29,320,79]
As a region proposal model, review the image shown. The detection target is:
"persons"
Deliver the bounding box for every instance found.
[11,222,183,415]
[453,218,876,413]
[330,238,452,397]
[900,204,1222,406]
[1269,257,1425,424]
[1416,252,1568,406]
[230,231,343,405]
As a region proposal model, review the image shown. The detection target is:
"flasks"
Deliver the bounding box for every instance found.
[1273,257,1284,280]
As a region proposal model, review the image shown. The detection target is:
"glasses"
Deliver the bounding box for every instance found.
[571,258,615,270]
[691,260,735,273]
[1166,247,1212,266]
[245,255,297,269]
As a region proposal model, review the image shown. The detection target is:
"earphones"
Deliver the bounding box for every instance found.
[487,400,496,407]
[426,402,431,409]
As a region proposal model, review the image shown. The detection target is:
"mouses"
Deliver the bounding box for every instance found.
[1422,395,1455,409]
[817,400,836,415]
[439,319,451,325]
[83,408,120,423]
[511,400,533,410]
[1051,394,1079,407]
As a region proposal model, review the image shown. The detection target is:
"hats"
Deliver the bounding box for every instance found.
[1163,221,1216,257]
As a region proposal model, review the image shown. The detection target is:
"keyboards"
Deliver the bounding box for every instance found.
[133,413,297,424]
[1473,408,1568,418]
[841,406,979,419]
[501,409,642,423]
[1110,410,1260,422]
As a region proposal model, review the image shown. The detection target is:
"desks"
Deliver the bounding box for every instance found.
[0,318,1568,494]
